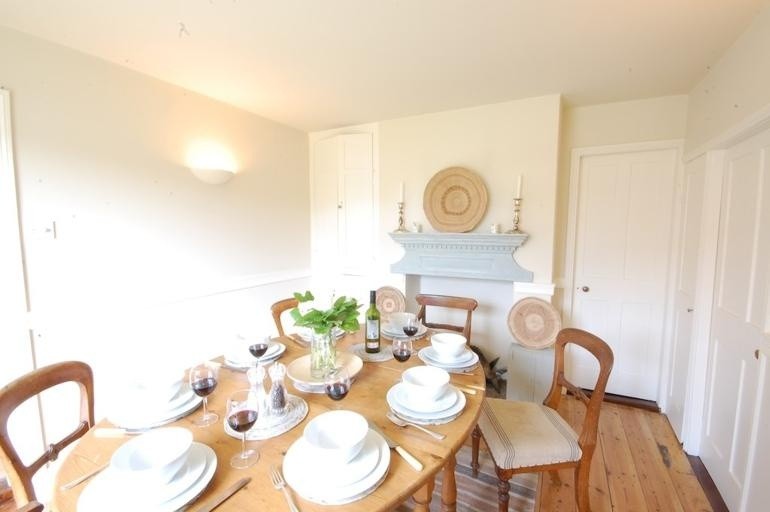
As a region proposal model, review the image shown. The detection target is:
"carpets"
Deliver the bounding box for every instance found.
[391,437,549,512]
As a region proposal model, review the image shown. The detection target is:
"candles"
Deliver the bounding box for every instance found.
[515,173,524,200]
[398,178,405,205]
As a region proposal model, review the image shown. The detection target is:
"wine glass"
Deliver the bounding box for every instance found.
[320,363,352,409]
[249,334,269,362]
[402,317,420,357]
[226,389,261,470]
[190,361,220,428]
[281,431,391,503]
[390,336,413,372]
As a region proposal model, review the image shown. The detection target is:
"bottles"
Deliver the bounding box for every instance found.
[246,360,290,421]
[365,290,381,354]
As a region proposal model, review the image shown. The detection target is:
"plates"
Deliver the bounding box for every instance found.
[223,340,287,370]
[287,352,362,395]
[296,326,348,341]
[125,386,202,430]
[75,440,219,512]
[380,323,428,339]
[418,345,479,368]
[385,381,467,418]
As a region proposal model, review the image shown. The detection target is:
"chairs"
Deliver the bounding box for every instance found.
[470,327,616,512]
[413,293,479,346]
[1,360,96,511]
[269,297,302,339]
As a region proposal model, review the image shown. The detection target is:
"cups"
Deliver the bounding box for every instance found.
[490,222,500,232]
[410,224,420,231]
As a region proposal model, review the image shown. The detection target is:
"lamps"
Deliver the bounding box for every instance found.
[191,166,237,187]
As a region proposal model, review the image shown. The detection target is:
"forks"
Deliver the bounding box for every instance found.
[269,468,300,510]
[384,411,447,440]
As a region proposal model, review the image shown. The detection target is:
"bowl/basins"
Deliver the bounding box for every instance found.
[401,365,451,403]
[388,311,416,331]
[109,424,193,491]
[430,332,469,357]
[302,408,369,464]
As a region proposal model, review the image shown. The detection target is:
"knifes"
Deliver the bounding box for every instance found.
[369,420,426,472]
[287,334,308,348]
[193,475,256,512]
